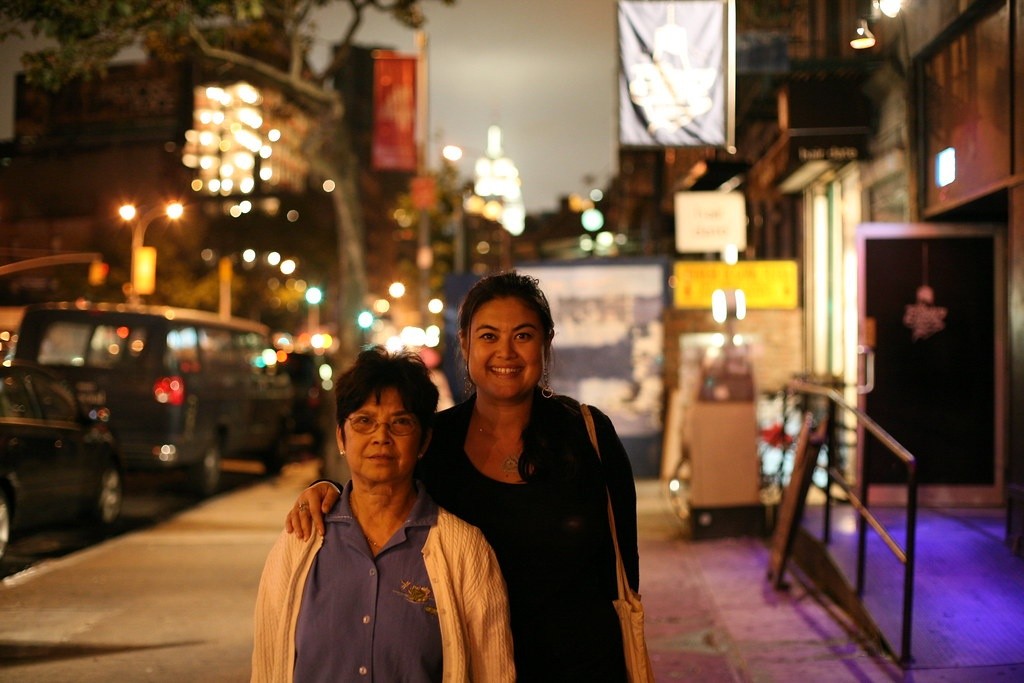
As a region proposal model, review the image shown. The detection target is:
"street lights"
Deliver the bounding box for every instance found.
[118,199,184,297]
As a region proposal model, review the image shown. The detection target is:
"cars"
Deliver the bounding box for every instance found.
[0,356,125,562]
[275,344,335,443]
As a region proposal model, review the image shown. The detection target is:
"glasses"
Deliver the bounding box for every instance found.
[345,414,421,435]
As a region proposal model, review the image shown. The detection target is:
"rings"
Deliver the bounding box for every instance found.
[299,503,308,510]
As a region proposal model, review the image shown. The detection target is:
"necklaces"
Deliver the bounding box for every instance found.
[479,424,521,478]
[365,536,381,549]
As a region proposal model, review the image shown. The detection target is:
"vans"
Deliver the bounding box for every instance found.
[9,302,295,497]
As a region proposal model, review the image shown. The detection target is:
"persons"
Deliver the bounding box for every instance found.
[250,345,516,683]
[286,269,640,683]
[666,343,770,491]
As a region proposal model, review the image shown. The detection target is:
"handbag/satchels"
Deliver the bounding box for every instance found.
[614,592,657,682]
